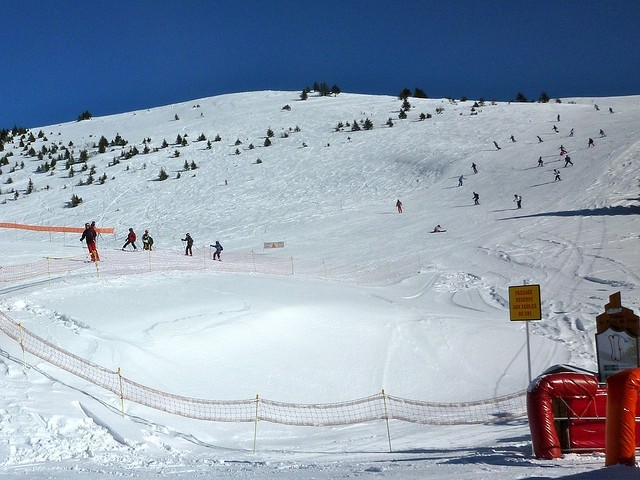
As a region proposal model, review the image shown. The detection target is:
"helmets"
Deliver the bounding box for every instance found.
[186,233,190,236]
[85,223,90,226]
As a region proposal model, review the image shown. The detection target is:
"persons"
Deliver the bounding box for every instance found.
[587,137,596,148]
[556,113,561,121]
[599,129,606,138]
[80,222,101,262]
[471,162,479,174]
[142,230,149,250]
[122,227,138,251]
[210,241,223,261]
[608,107,614,114]
[395,198,404,214]
[569,127,575,137]
[458,175,467,187]
[472,191,480,205]
[181,232,194,256]
[563,155,574,167]
[493,140,501,150]
[510,134,517,143]
[552,124,560,133]
[537,135,543,143]
[430,223,447,233]
[553,168,562,181]
[593,103,601,111]
[91,221,97,231]
[147,232,154,251]
[537,156,544,167]
[512,193,522,209]
[557,144,568,155]
